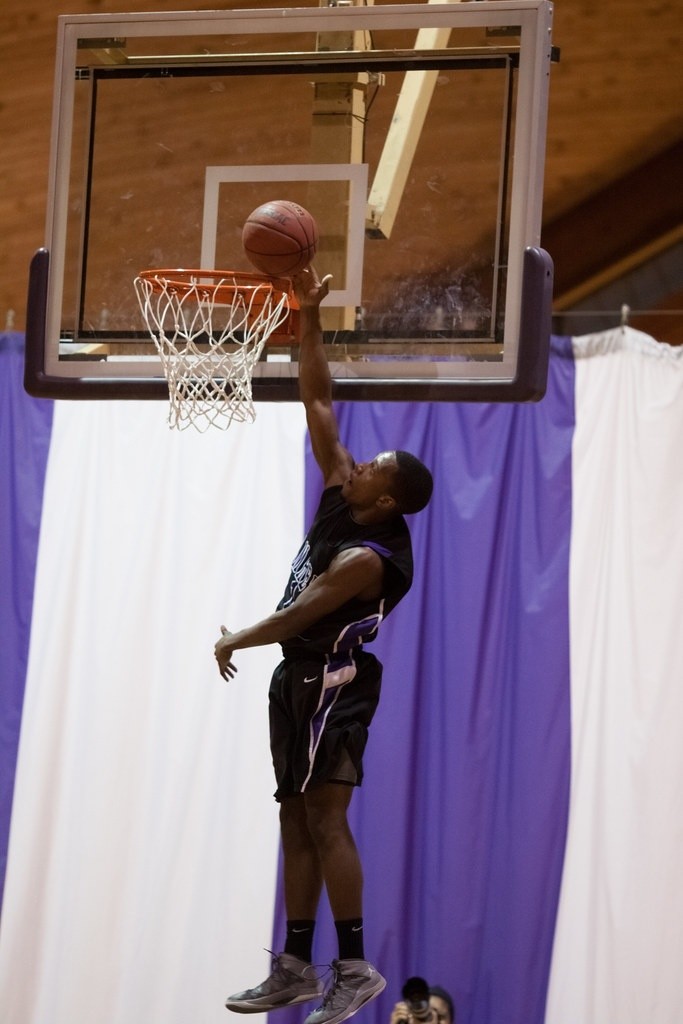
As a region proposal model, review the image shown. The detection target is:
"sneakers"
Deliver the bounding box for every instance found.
[301,958,387,1024]
[225,948,326,1014]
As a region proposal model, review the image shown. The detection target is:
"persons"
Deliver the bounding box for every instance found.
[215,263,434,1024]
[389,985,455,1024]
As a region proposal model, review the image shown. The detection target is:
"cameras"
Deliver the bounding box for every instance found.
[401,977,434,1021]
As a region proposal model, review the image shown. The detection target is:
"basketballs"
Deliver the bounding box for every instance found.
[241,198,319,277]
